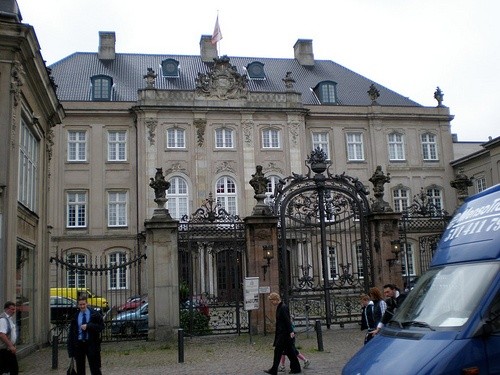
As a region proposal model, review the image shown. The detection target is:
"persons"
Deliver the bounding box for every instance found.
[264,292,302,375]
[0,301,20,375]
[280,314,311,372]
[67,295,105,375]
[358,294,376,331]
[369,287,387,333]
[370,284,406,337]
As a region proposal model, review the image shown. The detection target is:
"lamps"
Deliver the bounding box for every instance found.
[262,244,274,281]
[386,240,402,267]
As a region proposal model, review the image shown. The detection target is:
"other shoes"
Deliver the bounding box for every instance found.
[263,370,277,375]
[289,370,301,374]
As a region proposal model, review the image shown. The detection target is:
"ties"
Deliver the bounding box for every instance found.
[82,313,87,340]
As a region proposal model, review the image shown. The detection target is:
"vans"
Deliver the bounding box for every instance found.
[340,182,500,375]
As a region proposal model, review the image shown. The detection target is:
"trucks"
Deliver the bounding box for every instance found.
[49,288,107,317]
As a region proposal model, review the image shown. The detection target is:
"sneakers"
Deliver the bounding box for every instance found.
[278,366,287,372]
[304,359,312,369]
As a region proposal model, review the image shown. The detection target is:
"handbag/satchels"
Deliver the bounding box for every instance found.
[66,356,76,375]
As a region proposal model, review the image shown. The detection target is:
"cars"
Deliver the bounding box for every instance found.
[108,294,149,339]
[49,298,102,328]
